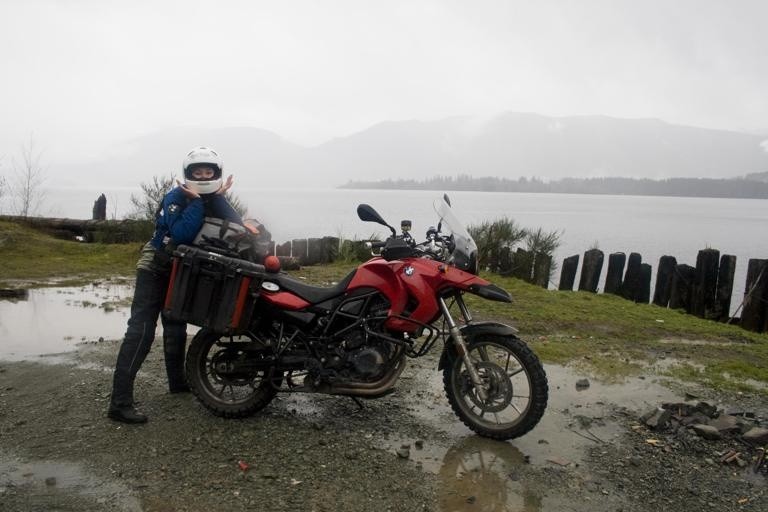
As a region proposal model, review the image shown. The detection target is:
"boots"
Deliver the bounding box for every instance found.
[108,333,157,423]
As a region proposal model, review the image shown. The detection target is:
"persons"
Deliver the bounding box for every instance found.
[108,148,242,425]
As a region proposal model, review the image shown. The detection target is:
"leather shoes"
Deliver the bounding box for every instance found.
[162,323,191,393]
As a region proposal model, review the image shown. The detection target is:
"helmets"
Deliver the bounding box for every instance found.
[183,148,222,194]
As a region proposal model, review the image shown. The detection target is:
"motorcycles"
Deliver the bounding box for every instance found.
[163,194,547,439]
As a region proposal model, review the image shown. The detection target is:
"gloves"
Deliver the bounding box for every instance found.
[198,233,255,260]
